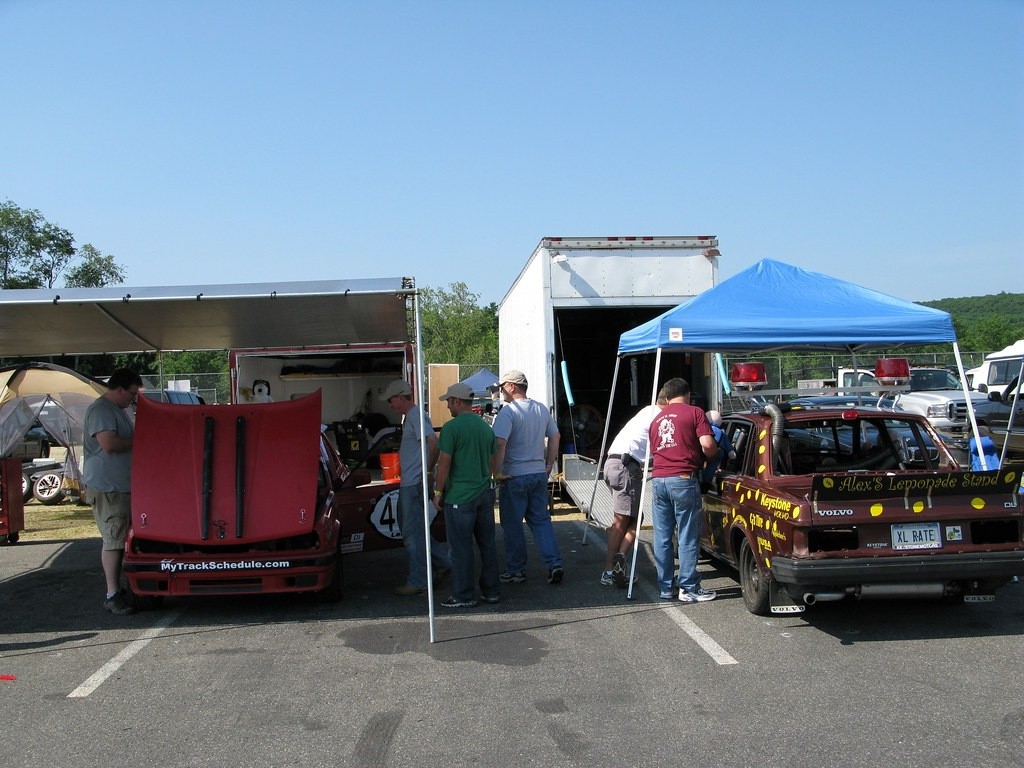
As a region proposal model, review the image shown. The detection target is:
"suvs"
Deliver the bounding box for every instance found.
[670,369,1024,615]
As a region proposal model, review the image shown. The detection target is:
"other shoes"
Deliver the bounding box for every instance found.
[395,582,427,596]
[699,481,709,494]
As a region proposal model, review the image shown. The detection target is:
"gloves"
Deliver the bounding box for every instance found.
[728,450,736,459]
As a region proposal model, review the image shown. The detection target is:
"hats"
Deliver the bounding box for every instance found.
[439,383,475,401]
[377,380,412,401]
[704,410,722,428]
[493,370,528,387]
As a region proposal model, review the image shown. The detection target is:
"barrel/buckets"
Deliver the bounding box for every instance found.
[380,453,400,479]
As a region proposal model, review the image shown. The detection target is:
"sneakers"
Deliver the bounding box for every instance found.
[660,584,679,599]
[441,596,477,608]
[103,592,136,615]
[600,571,637,586]
[678,585,716,601]
[480,592,497,603]
[548,565,563,584]
[611,552,627,588]
[498,571,527,583]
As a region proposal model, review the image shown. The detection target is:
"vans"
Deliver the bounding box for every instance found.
[134,389,208,405]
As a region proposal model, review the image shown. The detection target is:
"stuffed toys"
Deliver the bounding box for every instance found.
[247,378,274,404]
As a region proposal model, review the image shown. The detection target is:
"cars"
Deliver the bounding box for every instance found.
[4,416,51,462]
[121,387,447,612]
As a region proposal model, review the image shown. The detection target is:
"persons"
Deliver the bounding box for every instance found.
[79,367,142,613]
[377,379,439,597]
[432,383,502,608]
[648,378,717,603]
[490,369,564,588]
[698,410,736,495]
[600,388,668,587]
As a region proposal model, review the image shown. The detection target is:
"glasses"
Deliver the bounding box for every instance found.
[501,383,517,393]
[387,391,404,403]
[120,385,137,398]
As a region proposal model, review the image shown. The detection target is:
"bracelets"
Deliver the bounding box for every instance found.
[434,489,443,496]
[495,472,502,479]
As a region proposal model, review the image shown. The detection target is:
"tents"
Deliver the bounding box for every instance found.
[0,360,110,505]
[459,367,500,397]
[581,257,994,596]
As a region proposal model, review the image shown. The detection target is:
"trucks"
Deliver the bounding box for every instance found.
[227,341,426,487]
[797,340,1024,439]
[495,234,723,532]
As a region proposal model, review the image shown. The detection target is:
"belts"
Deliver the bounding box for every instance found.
[609,454,639,465]
